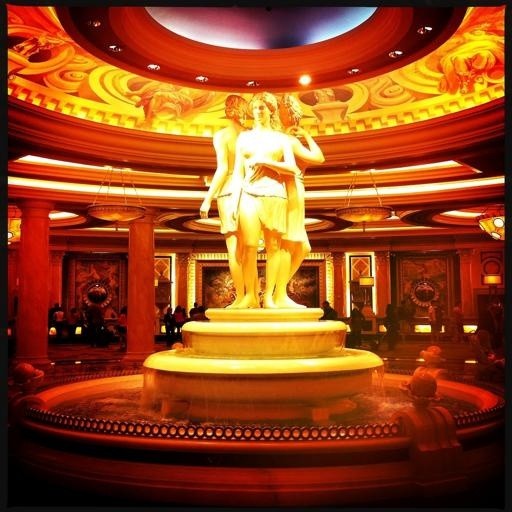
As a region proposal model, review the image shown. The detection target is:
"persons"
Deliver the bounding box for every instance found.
[48,302,206,348]
[384,303,401,353]
[199,94,261,310]
[349,308,367,346]
[228,89,299,311]
[399,295,465,344]
[271,93,328,309]
[321,300,339,321]
[475,298,505,352]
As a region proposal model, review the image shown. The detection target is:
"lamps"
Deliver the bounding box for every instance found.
[85,166,148,232]
[336,165,393,224]
